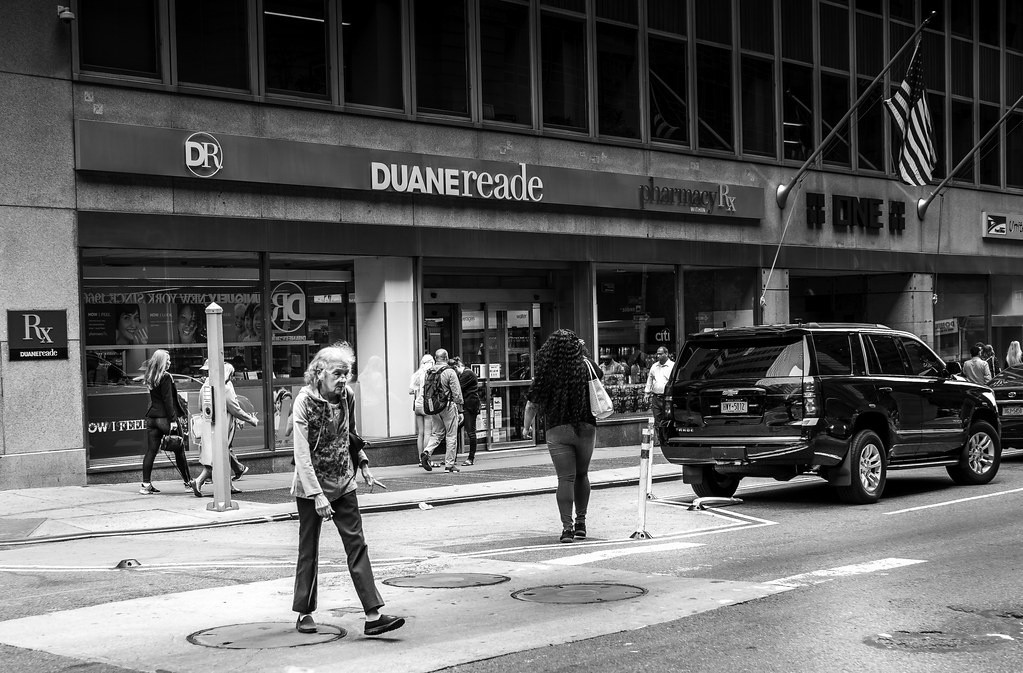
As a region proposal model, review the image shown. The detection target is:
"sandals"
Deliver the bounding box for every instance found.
[461,460,474,466]
[442,459,457,464]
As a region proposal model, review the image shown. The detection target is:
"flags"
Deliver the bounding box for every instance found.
[884,33,937,186]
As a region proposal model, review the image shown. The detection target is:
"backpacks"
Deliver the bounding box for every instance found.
[423,365,452,415]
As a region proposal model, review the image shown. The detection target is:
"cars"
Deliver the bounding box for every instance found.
[133,373,256,417]
[84,353,189,451]
[986,363,1023,452]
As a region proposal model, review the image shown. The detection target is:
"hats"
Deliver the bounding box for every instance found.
[199,358,209,370]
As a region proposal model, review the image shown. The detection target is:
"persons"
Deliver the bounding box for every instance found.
[140,348,195,495]
[173,302,210,373]
[963,342,1001,385]
[781,397,796,423]
[289,340,405,636]
[522,329,604,543]
[599,349,647,375]
[1003,341,1023,371]
[115,302,154,373]
[233,301,263,370]
[190,360,258,497]
[407,348,481,472]
[643,347,675,447]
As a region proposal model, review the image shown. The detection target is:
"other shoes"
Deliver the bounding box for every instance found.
[575,525,587,538]
[232,466,249,481]
[560,528,574,543]
[184,482,194,492]
[419,460,440,467]
[205,479,213,484]
[296,615,318,633]
[445,466,460,473]
[364,613,405,635]
[139,482,160,495]
[421,451,432,471]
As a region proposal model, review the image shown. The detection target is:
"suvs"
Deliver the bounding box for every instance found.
[659,322,1002,504]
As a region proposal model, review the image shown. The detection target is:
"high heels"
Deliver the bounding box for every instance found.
[190,480,204,496]
[230,486,242,493]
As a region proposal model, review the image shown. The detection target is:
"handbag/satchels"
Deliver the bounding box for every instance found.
[192,413,203,440]
[583,358,614,419]
[160,427,184,451]
[291,433,367,475]
